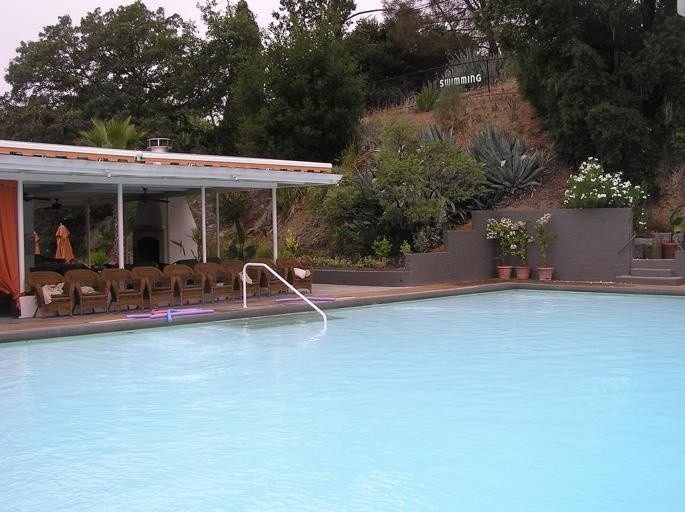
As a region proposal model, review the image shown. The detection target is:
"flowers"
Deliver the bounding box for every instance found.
[485,212,557,267]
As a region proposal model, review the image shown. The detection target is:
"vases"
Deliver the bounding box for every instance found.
[497,266,555,281]
[648,243,662,258]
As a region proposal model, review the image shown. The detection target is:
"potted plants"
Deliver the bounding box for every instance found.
[663,206,685,259]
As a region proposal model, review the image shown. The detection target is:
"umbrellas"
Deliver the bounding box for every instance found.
[55,222,75,265]
[29,230,41,255]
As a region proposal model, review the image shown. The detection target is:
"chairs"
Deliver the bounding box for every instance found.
[28,256,313,319]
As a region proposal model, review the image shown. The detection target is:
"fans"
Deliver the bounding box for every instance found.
[126,187,170,204]
[23,191,72,212]
